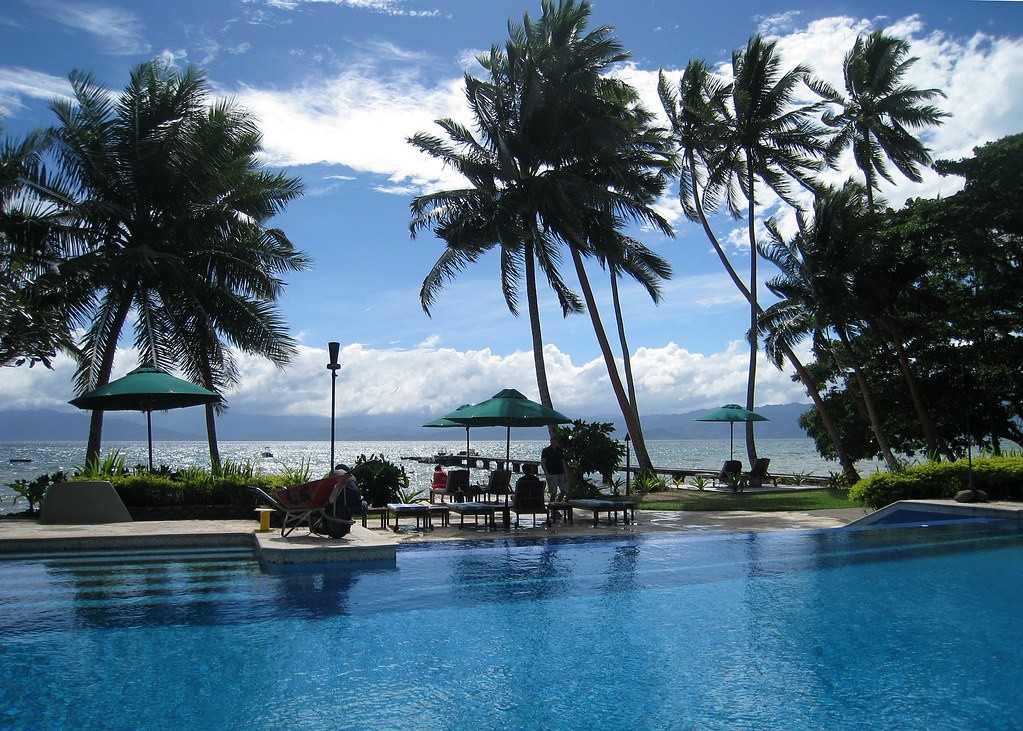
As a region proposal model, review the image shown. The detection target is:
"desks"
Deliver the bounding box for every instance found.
[475,486,487,502]
[485,504,513,527]
[615,502,637,522]
[362,506,387,529]
[546,502,573,524]
[425,505,450,528]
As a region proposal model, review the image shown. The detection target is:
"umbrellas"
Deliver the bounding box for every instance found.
[423,404,471,456]
[67,362,221,470]
[444,388,574,467]
[693,404,770,460]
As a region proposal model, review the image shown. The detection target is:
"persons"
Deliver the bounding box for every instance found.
[541,437,570,517]
[322,465,367,526]
[518,463,539,481]
[430,465,449,503]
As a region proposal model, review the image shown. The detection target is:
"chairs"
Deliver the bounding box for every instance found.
[693,458,770,488]
[387,470,637,528]
